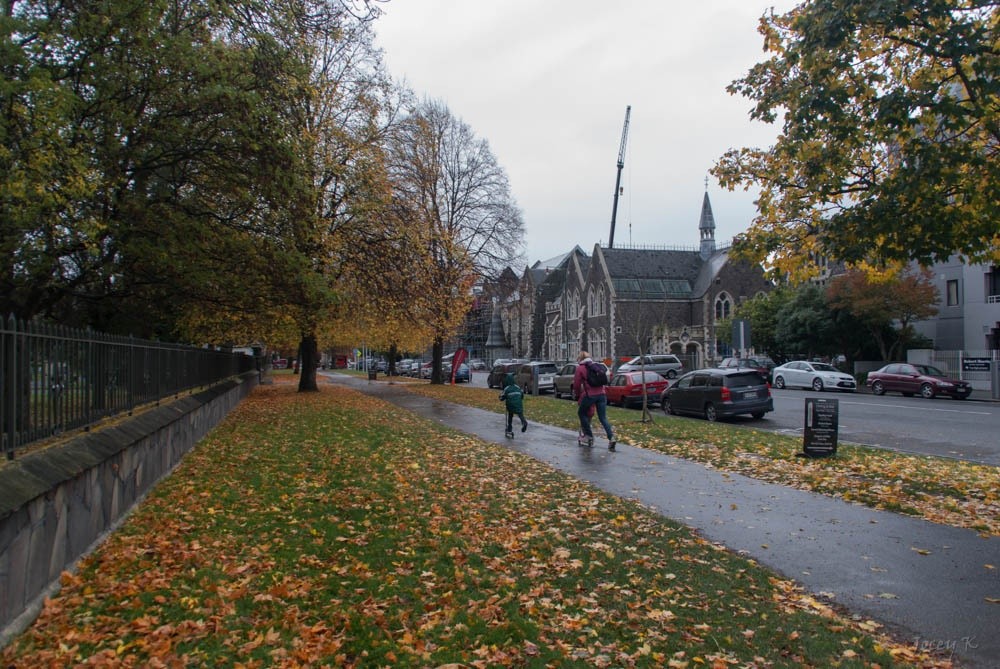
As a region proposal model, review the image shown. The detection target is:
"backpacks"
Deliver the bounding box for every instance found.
[579,360,608,387]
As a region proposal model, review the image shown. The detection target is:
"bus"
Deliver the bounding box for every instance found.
[335,354,349,369]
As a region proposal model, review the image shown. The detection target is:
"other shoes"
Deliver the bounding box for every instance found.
[521,421,527,432]
[505,425,512,432]
[579,435,588,442]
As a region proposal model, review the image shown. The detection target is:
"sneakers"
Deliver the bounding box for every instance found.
[608,437,616,450]
[589,439,595,448]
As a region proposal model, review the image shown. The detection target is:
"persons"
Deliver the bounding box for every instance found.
[573,352,616,450]
[498,373,527,433]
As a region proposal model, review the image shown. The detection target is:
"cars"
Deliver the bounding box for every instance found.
[866,362,973,400]
[350,357,474,384]
[659,368,773,419]
[493,359,512,367]
[513,358,530,364]
[467,360,487,368]
[605,371,671,409]
[770,361,860,391]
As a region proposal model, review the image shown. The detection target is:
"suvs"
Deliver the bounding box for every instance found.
[719,358,773,386]
[553,363,612,401]
[487,363,523,390]
[617,355,684,379]
[518,361,561,394]
